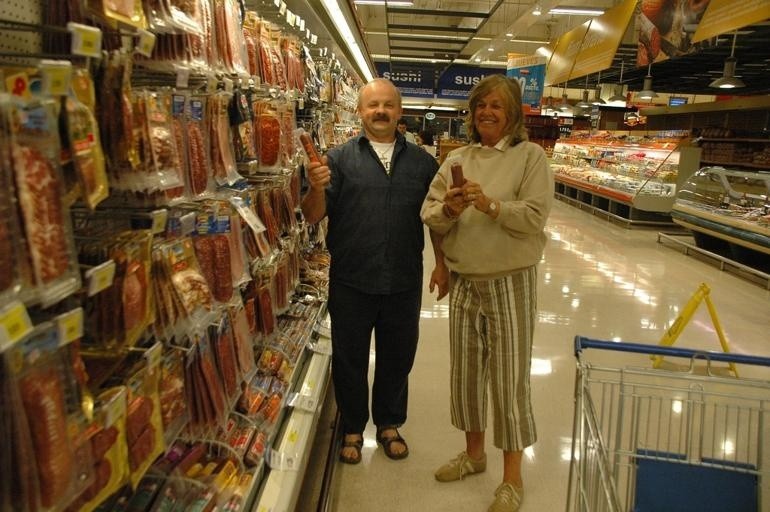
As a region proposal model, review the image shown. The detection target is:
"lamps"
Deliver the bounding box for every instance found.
[634,63,661,99]
[707,28,747,88]
[549,84,563,112]
[574,74,593,108]
[541,85,557,110]
[558,80,573,109]
[607,59,630,102]
[588,71,606,104]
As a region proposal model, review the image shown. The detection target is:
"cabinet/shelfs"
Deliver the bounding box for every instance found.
[545,129,702,224]
[669,164,770,272]
[639,92,769,170]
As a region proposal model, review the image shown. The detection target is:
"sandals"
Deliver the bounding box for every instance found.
[340,423,364,463]
[374,425,411,460]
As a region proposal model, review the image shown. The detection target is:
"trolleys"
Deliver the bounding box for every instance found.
[564,335,770,509]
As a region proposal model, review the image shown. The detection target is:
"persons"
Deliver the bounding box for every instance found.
[418,131,437,158]
[300,78,450,465]
[419,74,558,511]
[397,118,414,145]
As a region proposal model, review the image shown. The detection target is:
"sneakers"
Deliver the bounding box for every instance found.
[432,447,489,481]
[485,478,527,512]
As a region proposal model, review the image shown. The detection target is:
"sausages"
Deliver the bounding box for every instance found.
[300,132,330,187]
[450,161,464,187]
[110,334,311,512]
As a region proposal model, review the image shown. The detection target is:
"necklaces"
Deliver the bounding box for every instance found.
[369,140,396,162]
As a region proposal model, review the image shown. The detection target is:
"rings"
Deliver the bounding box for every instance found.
[472,200,474,204]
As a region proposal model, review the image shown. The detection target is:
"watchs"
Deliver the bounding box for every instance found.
[487,199,497,215]
